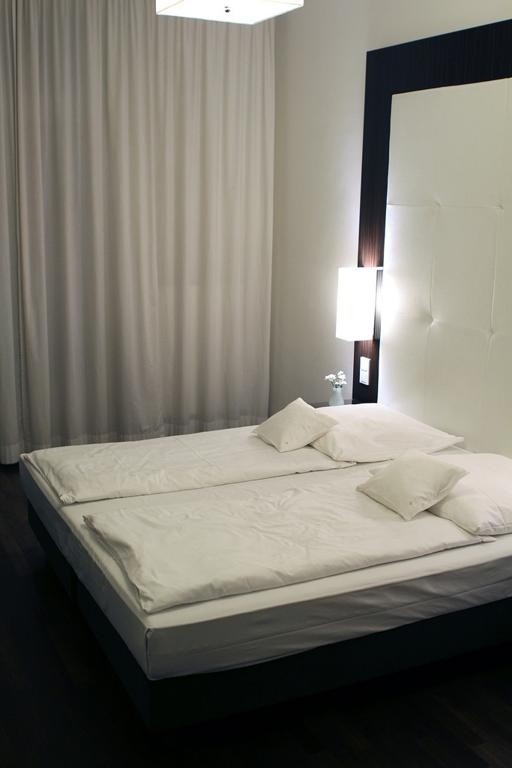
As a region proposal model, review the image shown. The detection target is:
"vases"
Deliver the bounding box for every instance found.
[329,387,344,406]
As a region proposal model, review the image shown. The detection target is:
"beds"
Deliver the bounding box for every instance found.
[18,401,512,735]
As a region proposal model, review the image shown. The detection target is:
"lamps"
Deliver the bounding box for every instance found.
[335,267,382,341]
[155,0,304,25]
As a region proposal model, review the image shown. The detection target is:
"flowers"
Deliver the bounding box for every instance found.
[324,370,347,388]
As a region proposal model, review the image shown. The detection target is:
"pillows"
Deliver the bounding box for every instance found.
[252,398,512,537]
